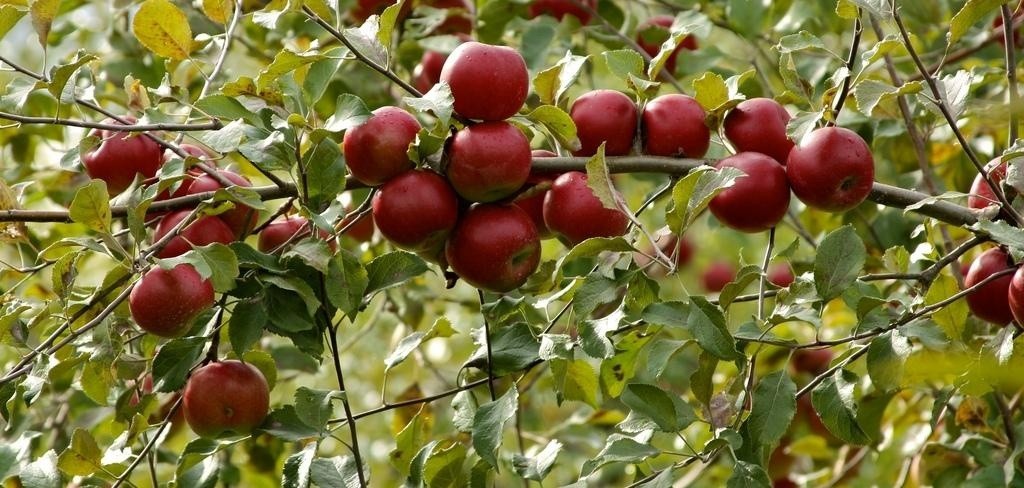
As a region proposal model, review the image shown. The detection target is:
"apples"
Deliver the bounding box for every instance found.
[968,155,1007,210]
[443,121,532,202]
[373,167,461,252]
[144,177,187,230]
[768,261,794,286]
[768,442,793,481]
[439,42,529,121]
[964,247,1022,328]
[542,171,630,250]
[184,360,270,440]
[798,395,833,437]
[154,207,239,259]
[569,88,640,157]
[186,172,260,237]
[704,263,733,291]
[80,117,161,196]
[513,150,567,240]
[528,1,598,26]
[1008,267,1023,328]
[411,32,477,96]
[707,152,791,234]
[350,2,472,38]
[993,4,1023,46]
[445,202,541,292]
[723,98,795,167]
[336,203,374,242]
[156,144,216,200]
[641,93,709,160]
[343,106,423,186]
[786,126,875,212]
[258,218,336,255]
[635,15,700,76]
[792,349,831,374]
[127,373,185,442]
[130,264,215,338]
[651,230,693,267]
